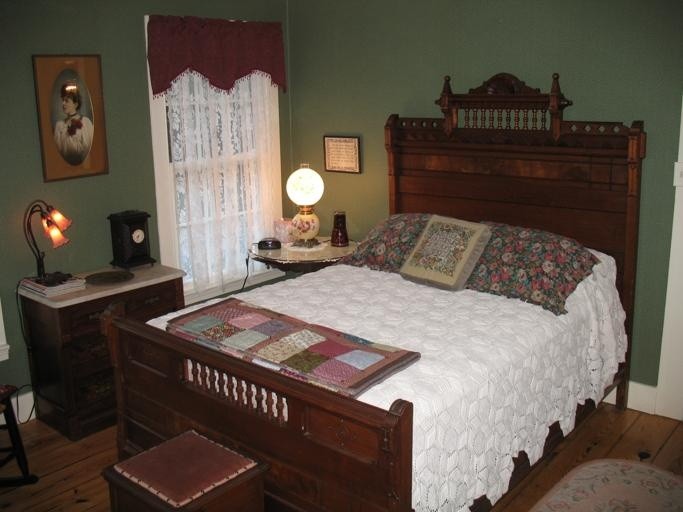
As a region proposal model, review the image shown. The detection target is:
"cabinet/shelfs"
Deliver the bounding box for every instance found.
[13,263,188,440]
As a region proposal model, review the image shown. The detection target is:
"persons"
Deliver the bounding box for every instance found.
[54,80,94,166]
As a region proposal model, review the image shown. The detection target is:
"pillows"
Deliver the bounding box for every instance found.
[335,211,601,317]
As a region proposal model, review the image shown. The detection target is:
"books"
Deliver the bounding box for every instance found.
[22,277,87,298]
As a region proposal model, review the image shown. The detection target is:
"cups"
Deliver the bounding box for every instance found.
[273,218,291,243]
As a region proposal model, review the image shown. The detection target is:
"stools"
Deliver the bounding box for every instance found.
[99,426,264,511]
[0,384,31,492]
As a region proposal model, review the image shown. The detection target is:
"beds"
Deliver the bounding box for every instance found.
[96,72,648,512]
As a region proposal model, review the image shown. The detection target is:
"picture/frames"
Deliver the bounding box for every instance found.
[322,135,362,175]
[32,52,110,182]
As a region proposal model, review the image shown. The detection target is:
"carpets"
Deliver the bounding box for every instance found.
[528,455,683,511]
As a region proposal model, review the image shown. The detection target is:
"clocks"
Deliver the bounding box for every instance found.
[106,209,157,268]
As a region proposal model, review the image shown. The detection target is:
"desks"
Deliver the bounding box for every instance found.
[249,235,356,278]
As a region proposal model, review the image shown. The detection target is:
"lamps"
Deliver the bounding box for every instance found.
[286,164,325,248]
[23,199,73,285]
